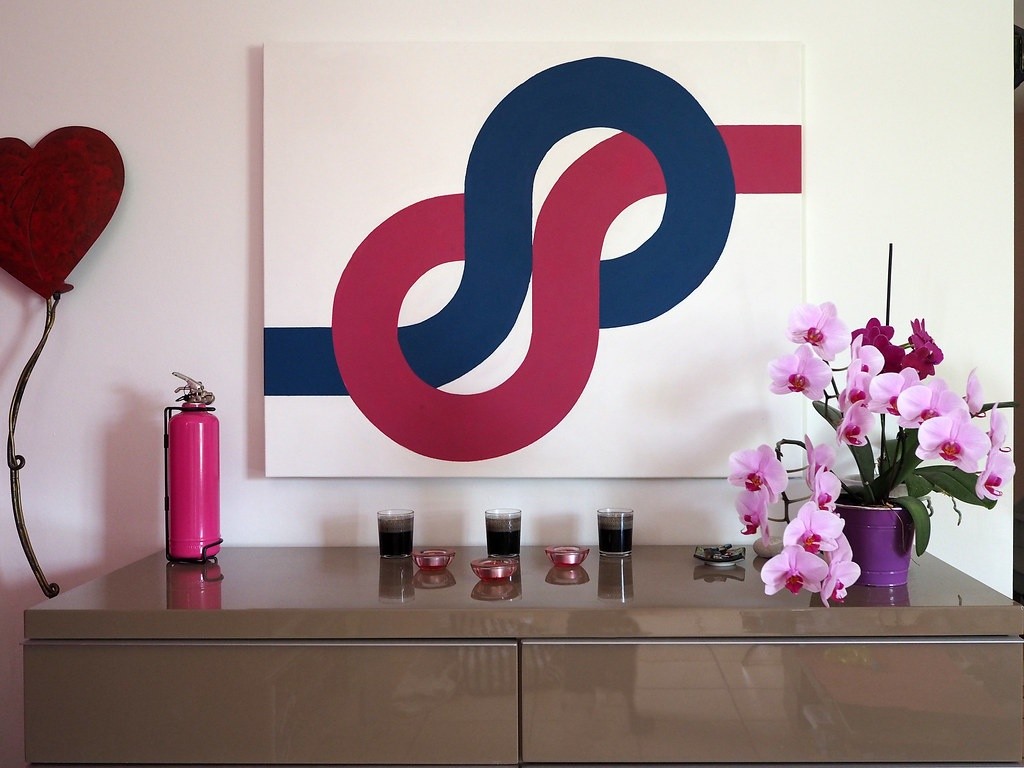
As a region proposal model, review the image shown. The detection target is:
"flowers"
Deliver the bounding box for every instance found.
[730,299,1020,608]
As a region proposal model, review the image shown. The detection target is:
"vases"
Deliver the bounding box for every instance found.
[834,504,913,588]
[808,584,911,608]
[842,474,908,497]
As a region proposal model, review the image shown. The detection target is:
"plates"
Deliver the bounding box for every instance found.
[693,546,746,567]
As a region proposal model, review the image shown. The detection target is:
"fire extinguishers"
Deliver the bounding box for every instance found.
[162,373,223,565]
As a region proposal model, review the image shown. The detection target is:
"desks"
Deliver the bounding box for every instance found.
[22,544,1024,768]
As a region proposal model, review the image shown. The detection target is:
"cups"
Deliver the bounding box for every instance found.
[484,508,522,558]
[597,555,634,603]
[597,507,634,557]
[378,556,414,604]
[376,508,415,559]
[488,555,522,601]
